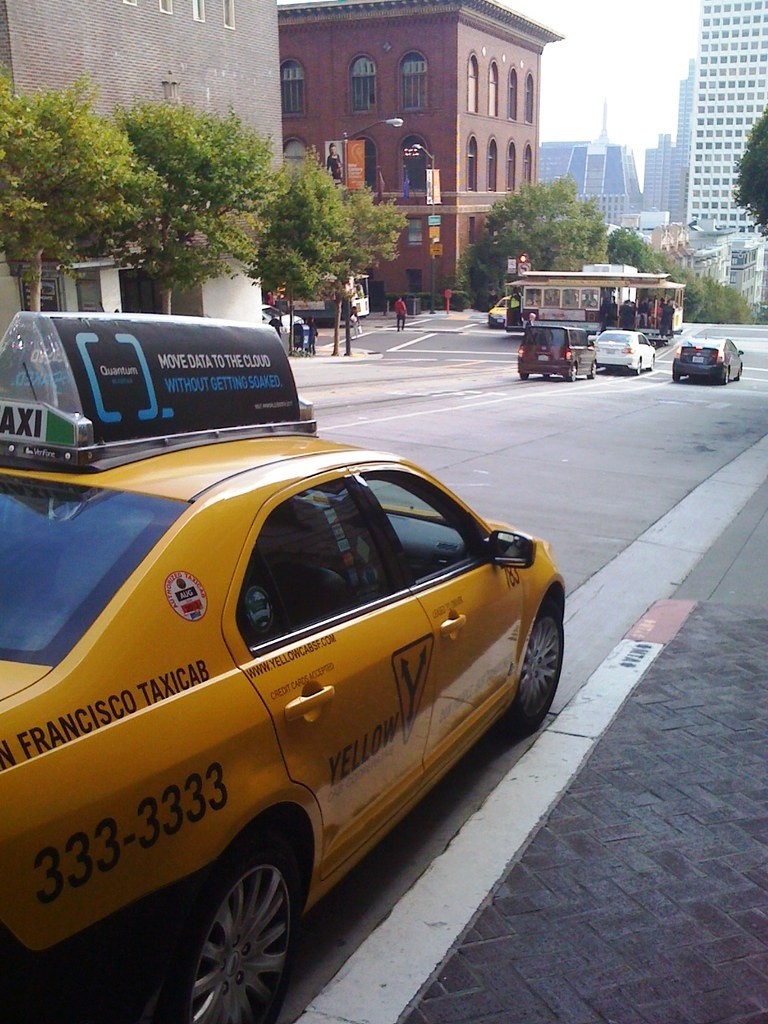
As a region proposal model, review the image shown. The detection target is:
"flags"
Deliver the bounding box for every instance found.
[378,171,385,201]
[404,169,409,199]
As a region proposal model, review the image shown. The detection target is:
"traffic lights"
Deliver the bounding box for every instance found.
[518,254,529,264]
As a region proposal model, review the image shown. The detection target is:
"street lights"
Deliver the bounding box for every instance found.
[343,118,403,356]
[412,143,436,314]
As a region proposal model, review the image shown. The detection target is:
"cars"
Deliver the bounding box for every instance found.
[594,329,656,375]
[673,338,744,386]
[0,313,565,1024]
[262,304,304,336]
[487,296,512,329]
[517,326,597,382]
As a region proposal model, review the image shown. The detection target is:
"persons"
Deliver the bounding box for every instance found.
[269,315,283,338]
[394,297,407,332]
[488,289,498,308]
[524,313,536,326]
[659,298,680,338]
[306,317,318,356]
[327,143,343,179]
[509,287,523,326]
[600,295,655,332]
[532,290,541,305]
[350,306,360,337]
[582,294,598,308]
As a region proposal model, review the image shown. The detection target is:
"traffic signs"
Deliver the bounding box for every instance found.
[430,244,442,256]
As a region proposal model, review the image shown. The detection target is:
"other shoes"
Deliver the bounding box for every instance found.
[659,335,661,339]
[663,335,667,339]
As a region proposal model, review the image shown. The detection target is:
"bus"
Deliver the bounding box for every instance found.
[506,271,687,346]
[267,273,369,323]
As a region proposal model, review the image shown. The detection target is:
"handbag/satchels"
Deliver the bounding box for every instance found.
[357,325,362,334]
